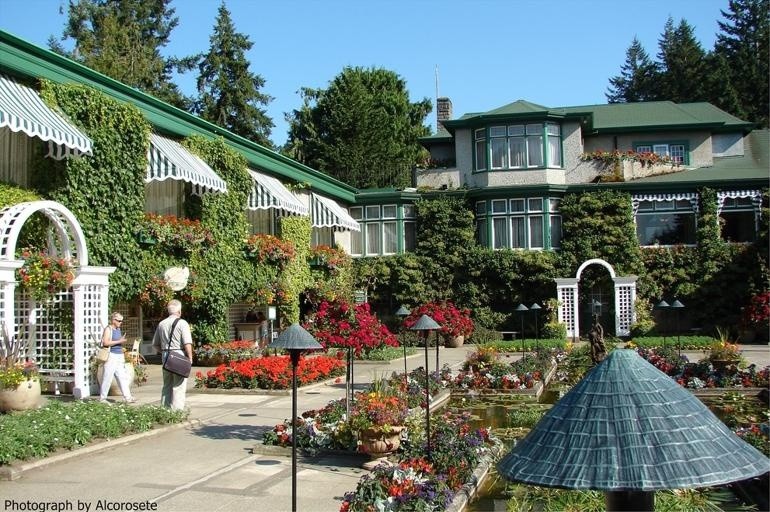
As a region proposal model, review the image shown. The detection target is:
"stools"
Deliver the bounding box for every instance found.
[496,330,519,340]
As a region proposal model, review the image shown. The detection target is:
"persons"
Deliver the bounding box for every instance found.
[98,310,140,404]
[149,299,194,409]
[589,312,605,361]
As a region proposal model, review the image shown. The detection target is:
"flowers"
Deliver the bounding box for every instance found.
[354,388,411,455]
[708,327,740,358]
[17,248,77,303]
[405,302,474,337]
[0,358,43,388]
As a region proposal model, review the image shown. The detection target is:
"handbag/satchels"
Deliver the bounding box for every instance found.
[96,348,110,360]
[163,351,192,378]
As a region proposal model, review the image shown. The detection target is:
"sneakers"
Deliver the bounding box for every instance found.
[126,397,139,403]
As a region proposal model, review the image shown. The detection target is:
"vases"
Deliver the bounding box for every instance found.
[1,379,41,411]
[444,334,463,345]
[97,363,135,394]
[357,425,403,468]
[711,361,737,377]
